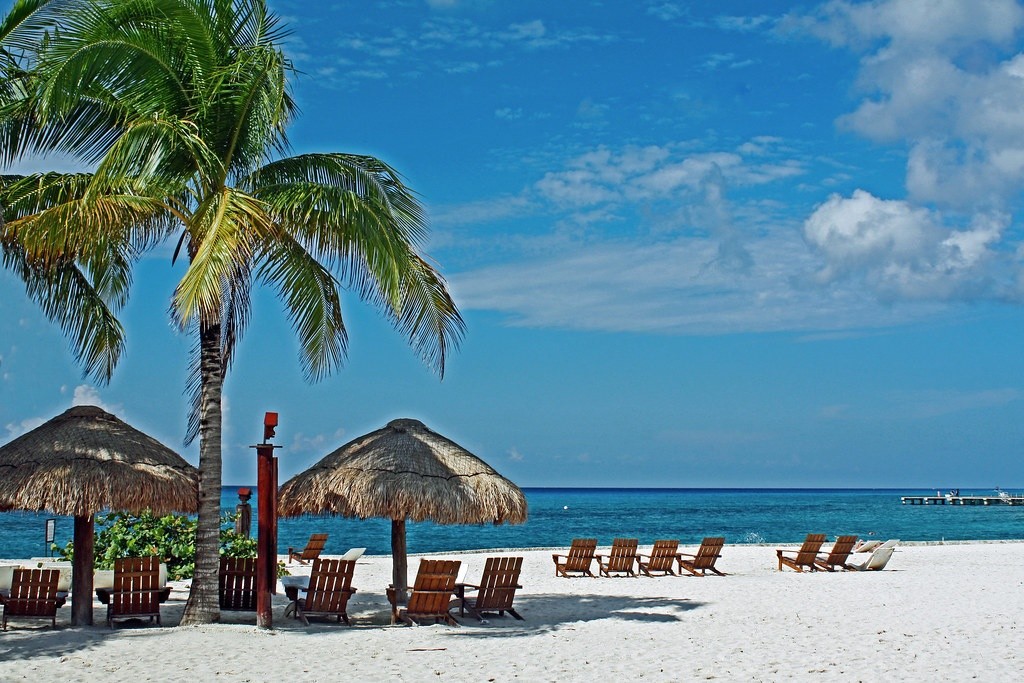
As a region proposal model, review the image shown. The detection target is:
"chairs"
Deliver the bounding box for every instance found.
[0,569,67,632]
[596,538,640,579]
[287,533,330,565]
[676,537,726,578]
[387,559,464,628]
[453,556,525,623]
[282,547,366,601]
[636,540,680,578]
[845,547,895,571]
[871,539,900,556]
[293,558,358,626]
[553,538,598,579]
[776,534,830,573]
[96,554,171,629]
[815,534,858,572]
[853,540,879,553]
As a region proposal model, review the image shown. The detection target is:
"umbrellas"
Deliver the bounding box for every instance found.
[0,405,199,624]
[277,417,528,629]
